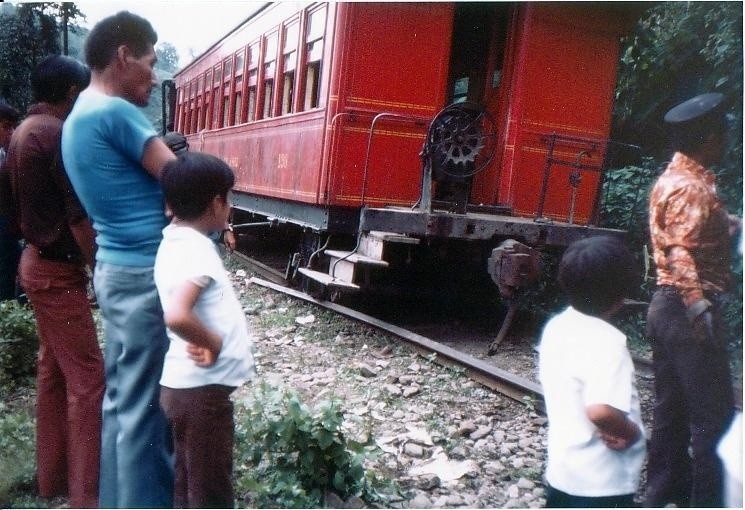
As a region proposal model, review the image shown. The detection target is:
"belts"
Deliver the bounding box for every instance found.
[37,245,81,263]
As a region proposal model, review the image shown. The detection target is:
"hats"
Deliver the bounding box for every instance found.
[663,92,736,135]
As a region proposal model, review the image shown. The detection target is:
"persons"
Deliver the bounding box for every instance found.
[6,53,106,508]
[538,227,651,508]
[635,86,744,508]
[163,130,236,255]
[0,101,29,304]
[153,148,256,510]
[61,12,179,509]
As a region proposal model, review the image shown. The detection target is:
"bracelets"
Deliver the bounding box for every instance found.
[223,226,234,233]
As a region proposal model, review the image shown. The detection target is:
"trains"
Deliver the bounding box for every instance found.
[160,1,659,355]
